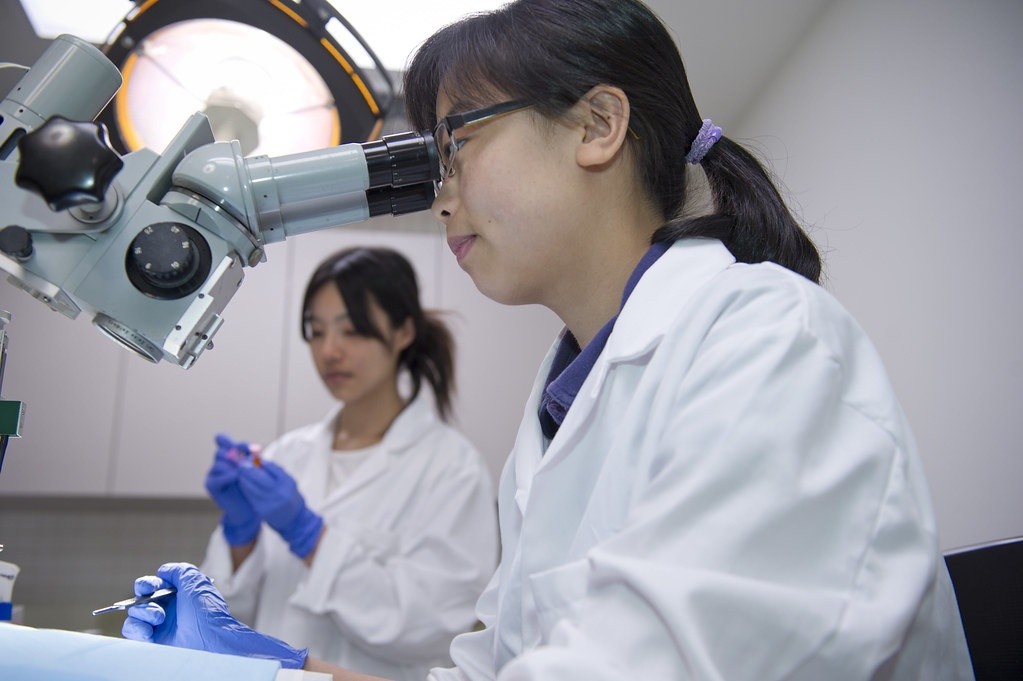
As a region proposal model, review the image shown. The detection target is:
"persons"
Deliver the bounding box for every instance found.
[199,247,498,681]
[121,0,978,681]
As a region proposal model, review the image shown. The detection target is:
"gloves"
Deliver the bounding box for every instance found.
[123,562,310,674]
[206,435,259,548]
[236,457,323,558]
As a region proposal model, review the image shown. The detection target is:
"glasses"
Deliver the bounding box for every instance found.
[432,94,642,195]
